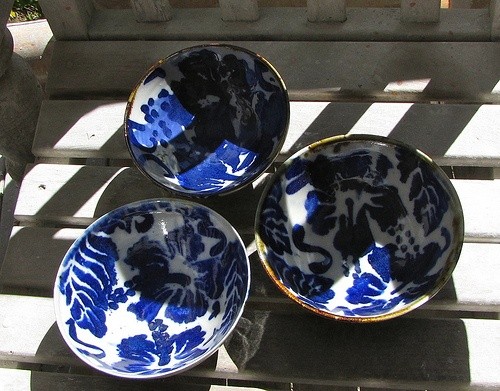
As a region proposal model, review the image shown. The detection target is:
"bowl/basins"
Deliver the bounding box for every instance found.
[123,43,290,199]
[253,133,465,324]
[54,199,250,380]
